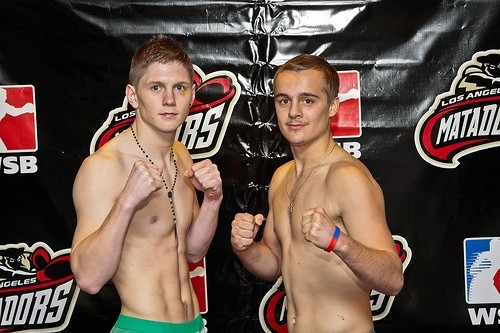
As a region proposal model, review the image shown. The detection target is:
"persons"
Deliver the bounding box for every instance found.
[232,54,403,333]
[70,40,223,333]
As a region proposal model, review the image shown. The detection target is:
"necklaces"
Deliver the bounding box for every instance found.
[324,226,340,253]
[285,144,338,212]
[130,125,178,238]
